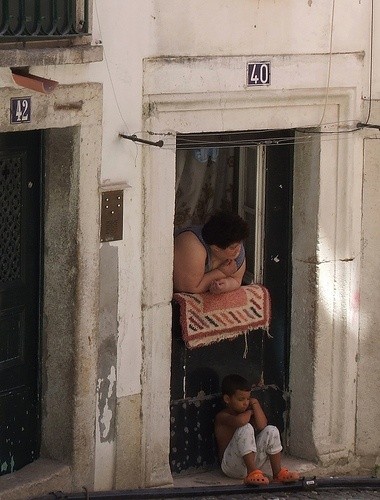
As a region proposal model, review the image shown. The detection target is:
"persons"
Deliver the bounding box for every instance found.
[173,212,249,295]
[214,374,299,485]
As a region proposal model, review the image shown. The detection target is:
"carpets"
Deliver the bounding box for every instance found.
[173,284,271,351]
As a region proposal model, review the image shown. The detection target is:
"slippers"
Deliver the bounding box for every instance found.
[244,470,269,485]
[273,469,299,481]
